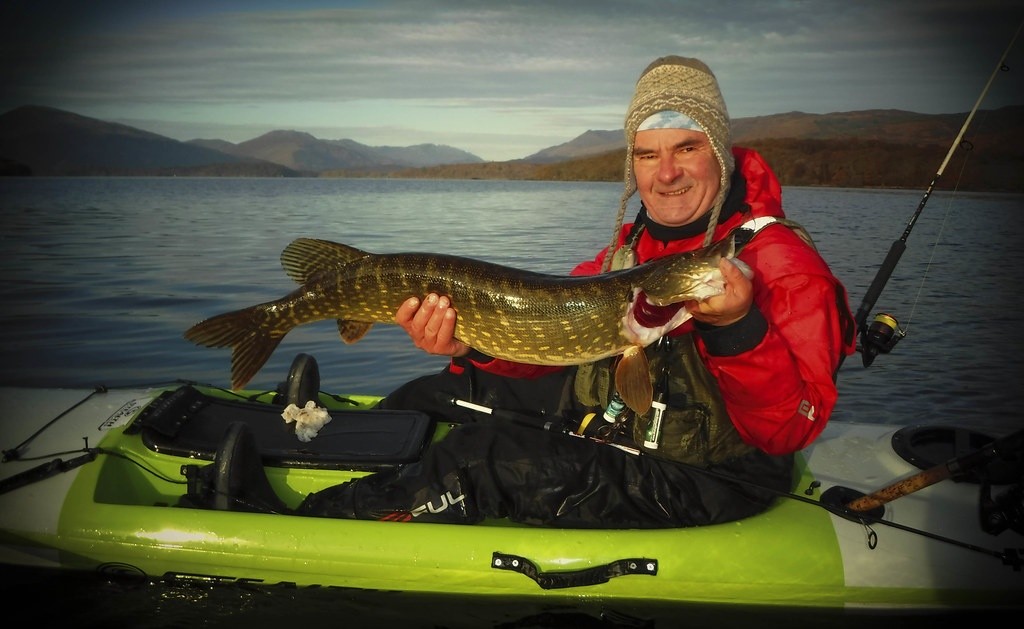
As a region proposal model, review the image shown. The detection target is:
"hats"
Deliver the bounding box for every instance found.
[601,55,735,273]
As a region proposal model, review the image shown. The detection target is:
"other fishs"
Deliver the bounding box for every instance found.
[185,226,755,391]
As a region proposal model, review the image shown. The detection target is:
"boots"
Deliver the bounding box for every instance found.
[286,353,328,409]
[214,422,358,520]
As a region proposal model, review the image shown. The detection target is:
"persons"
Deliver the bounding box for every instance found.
[215,53,858,523]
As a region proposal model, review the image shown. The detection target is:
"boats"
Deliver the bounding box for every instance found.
[1,350,1024,625]
